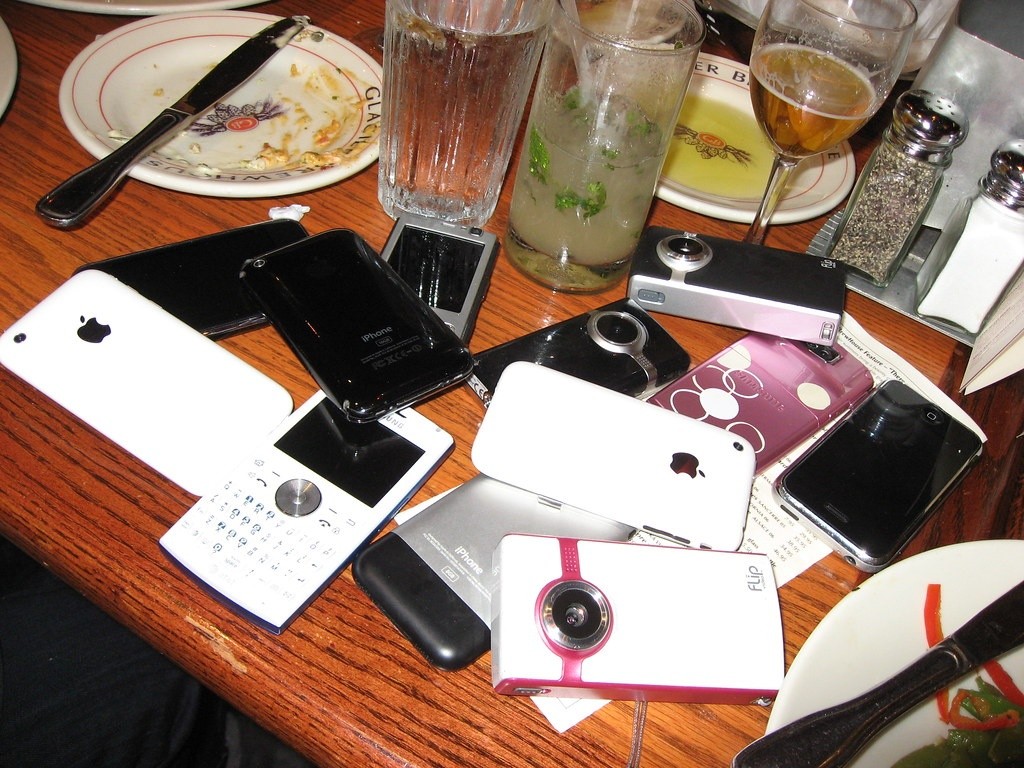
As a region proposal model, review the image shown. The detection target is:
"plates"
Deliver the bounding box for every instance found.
[654,53,856,225]
[766,538,1022,765]
[59,10,384,197]
[0,16,18,119]
[20,0,272,15]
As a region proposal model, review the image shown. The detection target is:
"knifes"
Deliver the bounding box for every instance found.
[36,16,311,230]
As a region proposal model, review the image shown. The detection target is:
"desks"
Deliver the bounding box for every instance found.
[0,0,1022,768]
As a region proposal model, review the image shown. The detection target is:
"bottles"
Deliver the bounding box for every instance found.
[915,139,1024,339]
[826,89,969,288]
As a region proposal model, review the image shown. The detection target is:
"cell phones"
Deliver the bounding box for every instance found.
[0,217,985,707]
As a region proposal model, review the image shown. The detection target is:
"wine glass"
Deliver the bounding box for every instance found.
[743,1,918,246]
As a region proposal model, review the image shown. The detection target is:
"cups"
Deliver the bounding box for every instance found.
[379,0,555,229]
[730,578,1023,768]
[501,0,706,293]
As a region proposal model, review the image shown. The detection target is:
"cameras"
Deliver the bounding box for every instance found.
[628,224,847,346]
[381,213,501,349]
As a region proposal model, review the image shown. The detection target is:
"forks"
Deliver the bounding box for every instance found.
[694,0,726,47]
